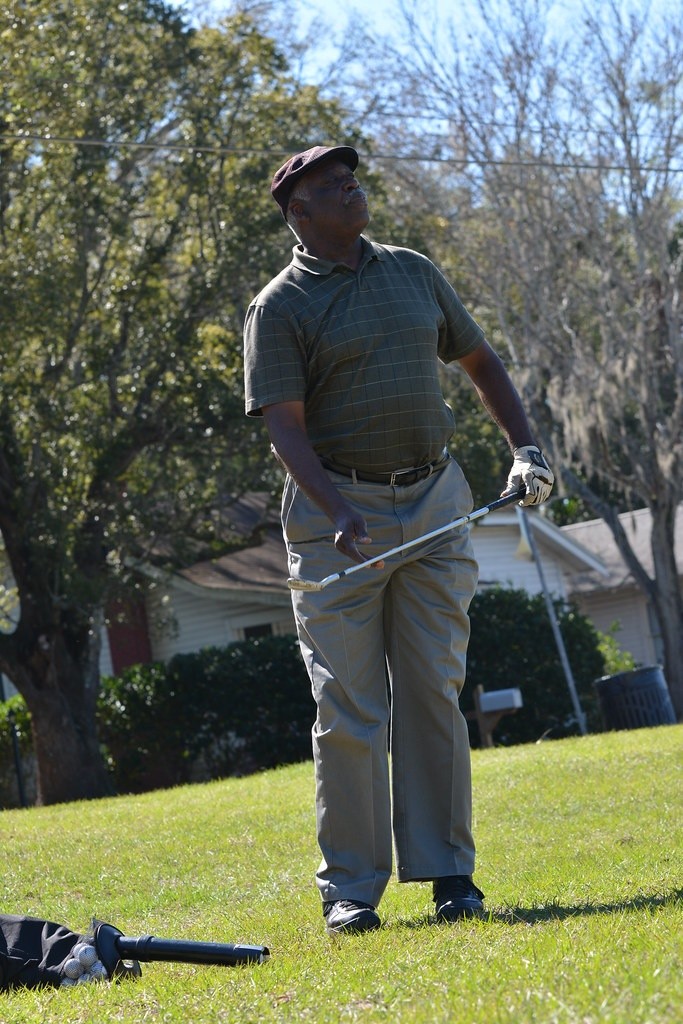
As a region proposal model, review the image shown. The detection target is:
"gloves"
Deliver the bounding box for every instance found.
[500,445,554,507]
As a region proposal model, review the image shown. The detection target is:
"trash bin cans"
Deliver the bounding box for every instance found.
[593,664,678,730]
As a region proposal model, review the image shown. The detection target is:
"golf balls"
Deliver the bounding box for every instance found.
[59,942,110,987]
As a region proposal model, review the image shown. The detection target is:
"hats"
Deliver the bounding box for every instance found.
[271,145,358,222]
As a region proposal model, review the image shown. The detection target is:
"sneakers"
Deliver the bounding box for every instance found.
[325,899,382,933]
[432,877,486,921]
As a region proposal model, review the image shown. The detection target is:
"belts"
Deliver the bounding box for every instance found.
[318,447,452,487]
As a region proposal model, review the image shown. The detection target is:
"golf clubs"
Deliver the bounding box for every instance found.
[284,482,526,594]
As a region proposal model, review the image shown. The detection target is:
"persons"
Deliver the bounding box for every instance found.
[242,145,555,937]
